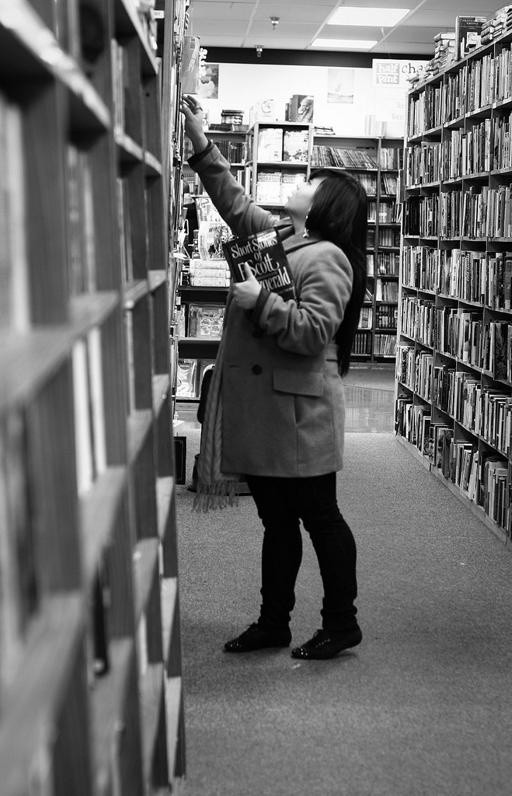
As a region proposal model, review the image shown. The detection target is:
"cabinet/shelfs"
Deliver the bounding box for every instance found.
[181,113,407,408]
[0,0,196,796]
[393,30,511,546]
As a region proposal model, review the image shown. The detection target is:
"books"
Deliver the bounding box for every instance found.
[406,7,511,92]
[402,295,511,383]
[395,340,511,457]
[394,394,511,531]
[402,115,511,238]
[402,245,512,313]
[172,94,407,360]
[2,0,215,796]
[409,48,511,137]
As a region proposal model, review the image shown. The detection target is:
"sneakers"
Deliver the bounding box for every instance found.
[226,622,292,651]
[291,627,362,660]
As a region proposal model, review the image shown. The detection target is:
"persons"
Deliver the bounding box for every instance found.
[173,89,371,665]
